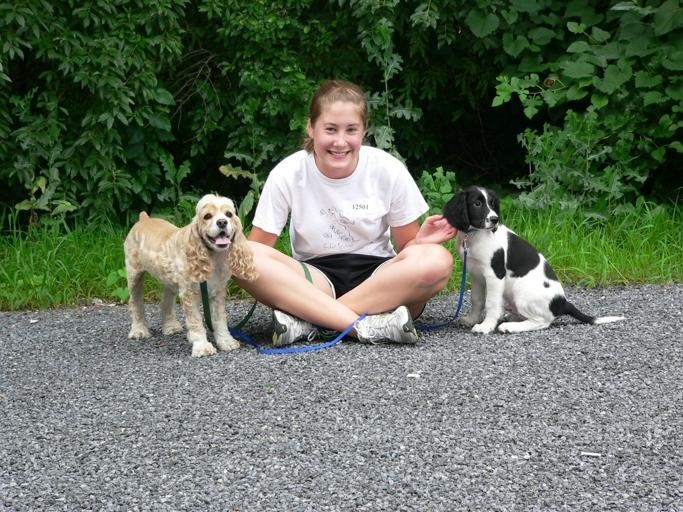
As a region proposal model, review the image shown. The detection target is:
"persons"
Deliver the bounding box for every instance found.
[225,77,459,346]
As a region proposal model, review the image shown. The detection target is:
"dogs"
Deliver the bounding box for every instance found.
[443,184,629,336]
[121,189,261,359]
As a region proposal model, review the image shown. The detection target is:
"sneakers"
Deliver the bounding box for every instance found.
[356,304,419,344]
[270,309,319,347]
